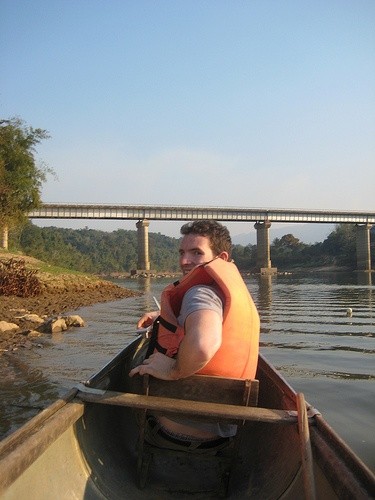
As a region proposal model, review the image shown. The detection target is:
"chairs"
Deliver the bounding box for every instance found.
[138,371,259,499]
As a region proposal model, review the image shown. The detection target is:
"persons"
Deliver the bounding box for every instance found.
[129,220,261,449]
